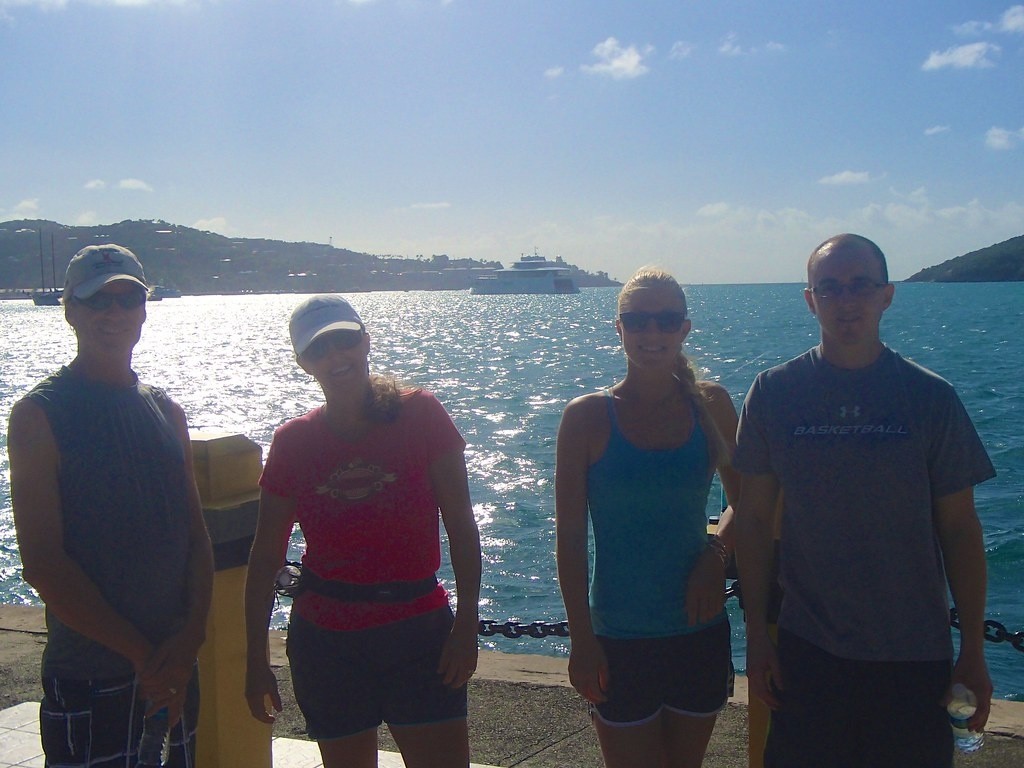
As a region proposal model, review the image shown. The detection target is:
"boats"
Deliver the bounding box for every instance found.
[471,254,580,295]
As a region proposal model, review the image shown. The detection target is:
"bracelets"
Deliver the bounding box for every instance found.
[708,536,731,570]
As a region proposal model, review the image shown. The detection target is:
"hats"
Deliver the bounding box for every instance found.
[287,298,367,357]
[63,243,151,307]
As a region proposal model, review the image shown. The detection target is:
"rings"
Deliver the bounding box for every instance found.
[169,688,177,694]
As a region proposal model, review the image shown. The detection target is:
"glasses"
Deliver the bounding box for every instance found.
[617,309,689,336]
[300,330,366,356]
[808,277,890,298]
[67,289,145,310]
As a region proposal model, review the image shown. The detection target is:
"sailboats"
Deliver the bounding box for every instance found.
[30,226,64,306]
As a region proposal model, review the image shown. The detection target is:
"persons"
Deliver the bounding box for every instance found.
[7,243,214,768]
[244,294,483,768]
[731,234,994,768]
[555,270,738,768]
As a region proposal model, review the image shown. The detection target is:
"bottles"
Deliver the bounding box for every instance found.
[274,565,302,596]
[139,659,178,765]
[948,683,985,753]
